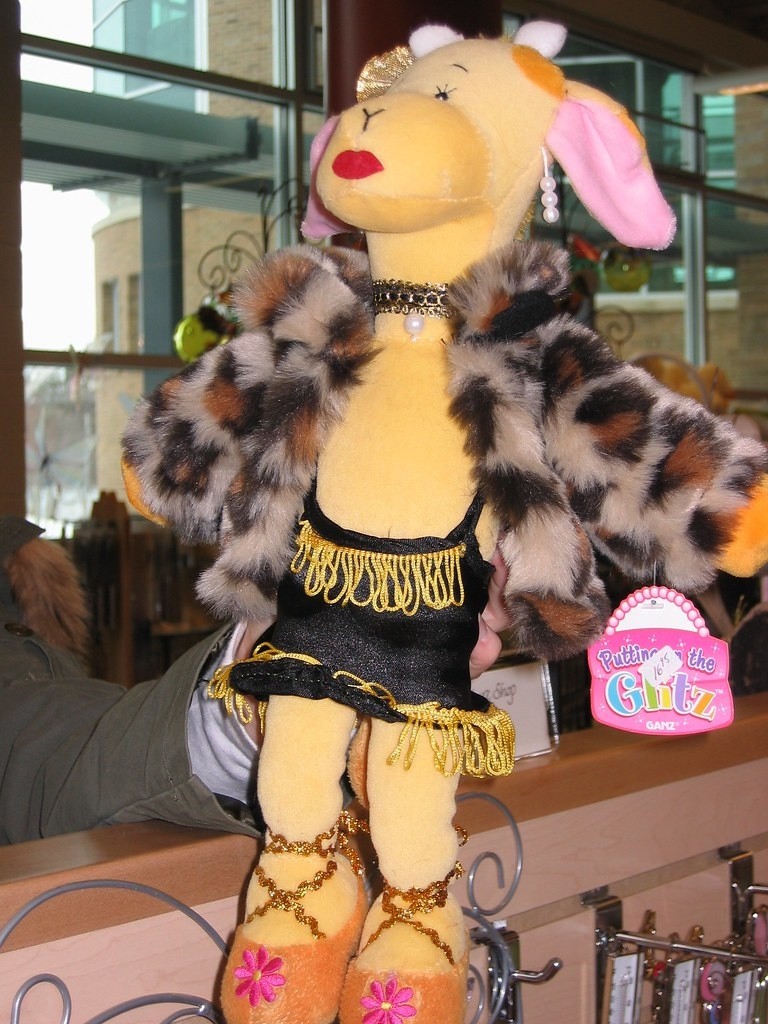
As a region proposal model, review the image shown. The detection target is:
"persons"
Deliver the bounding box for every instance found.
[0,506,507,845]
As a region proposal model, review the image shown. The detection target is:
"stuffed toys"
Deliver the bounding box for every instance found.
[123,21,768,1024]
